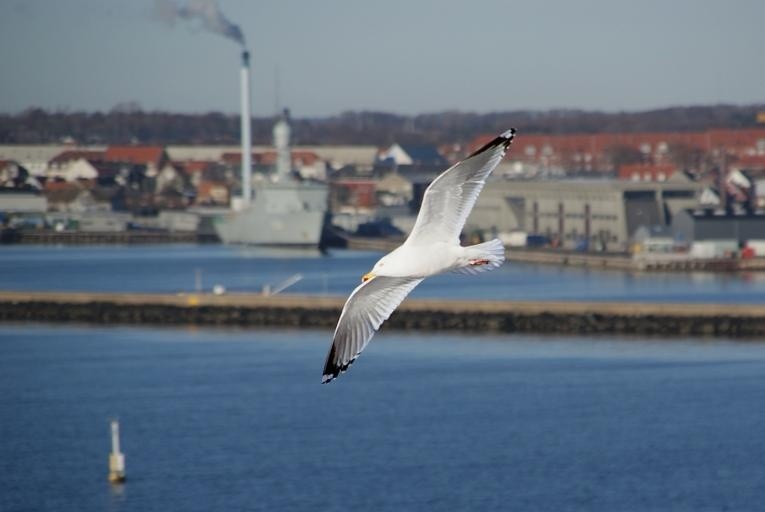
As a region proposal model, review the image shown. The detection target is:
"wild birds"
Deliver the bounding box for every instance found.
[321,127,518,385]
